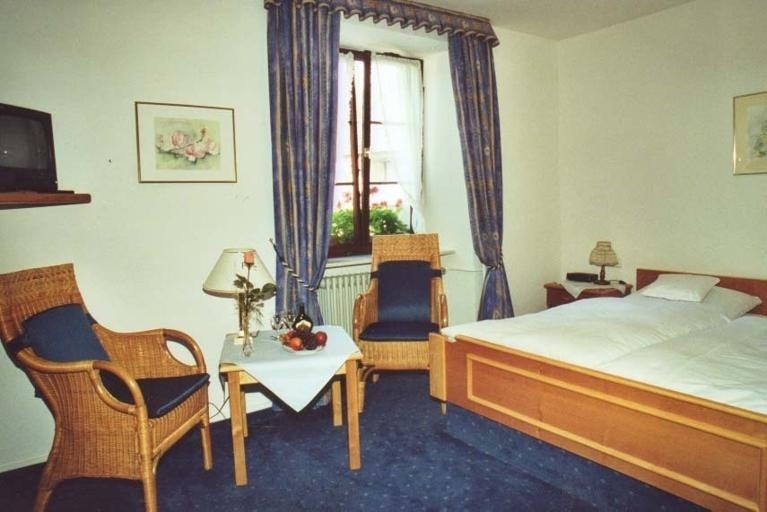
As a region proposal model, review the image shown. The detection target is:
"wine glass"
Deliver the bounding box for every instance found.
[271,308,297,344]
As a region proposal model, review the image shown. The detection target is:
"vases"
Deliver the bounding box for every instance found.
[239,322,250,358]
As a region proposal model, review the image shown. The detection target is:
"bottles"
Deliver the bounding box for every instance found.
[291,302,312,332]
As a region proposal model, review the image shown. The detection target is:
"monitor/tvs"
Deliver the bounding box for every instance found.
[0,102,58,193]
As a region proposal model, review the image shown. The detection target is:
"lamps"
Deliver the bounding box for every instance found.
[587,240,620,284]
[198,246,279,347]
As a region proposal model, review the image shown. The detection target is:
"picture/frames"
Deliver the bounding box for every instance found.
[130,98,238,189]
[729,89,766,177]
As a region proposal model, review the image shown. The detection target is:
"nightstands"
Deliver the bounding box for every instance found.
[544,278,630,311]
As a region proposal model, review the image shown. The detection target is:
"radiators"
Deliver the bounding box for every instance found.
[316,269,370,337]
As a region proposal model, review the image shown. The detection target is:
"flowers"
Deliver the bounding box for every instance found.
[234,249,277,327]
[153,126,220,168]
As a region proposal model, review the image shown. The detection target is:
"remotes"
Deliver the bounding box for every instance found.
[37,190,74,194]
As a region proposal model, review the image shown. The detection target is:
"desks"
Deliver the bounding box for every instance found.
[216,318,366,491]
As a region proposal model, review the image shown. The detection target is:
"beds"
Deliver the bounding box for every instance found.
[425,266,767,512]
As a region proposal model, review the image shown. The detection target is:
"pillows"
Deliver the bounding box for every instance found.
[624,281,763,323]
[643,272,722,304]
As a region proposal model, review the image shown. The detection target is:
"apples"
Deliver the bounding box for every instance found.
[315,331,327,345]
[291,337,303,350]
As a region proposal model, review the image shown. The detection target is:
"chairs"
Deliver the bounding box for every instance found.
[352,231,453,417]
[0,262,214,512]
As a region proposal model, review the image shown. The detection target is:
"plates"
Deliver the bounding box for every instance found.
[282,343,321,354]
